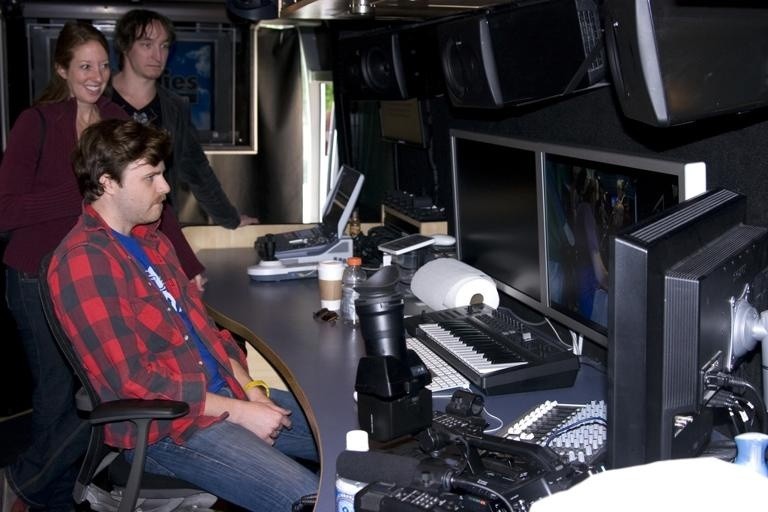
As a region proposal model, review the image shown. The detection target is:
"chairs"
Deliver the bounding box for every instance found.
[21,241,229,511]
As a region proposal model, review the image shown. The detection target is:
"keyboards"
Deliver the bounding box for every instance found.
[381,189,450,222]
[406,337,471,393]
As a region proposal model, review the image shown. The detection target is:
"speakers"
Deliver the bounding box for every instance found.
[362,23,447,101]
[600,0,768,152]
[437,0,611,109]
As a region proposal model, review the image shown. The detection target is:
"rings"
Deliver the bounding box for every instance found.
[273,431,280,438]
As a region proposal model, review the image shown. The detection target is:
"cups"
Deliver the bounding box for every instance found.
[316,260,344,311]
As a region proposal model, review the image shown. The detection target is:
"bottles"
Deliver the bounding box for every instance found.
[341,257,366,325]
[731,431,767,475]
[333,429,379,512]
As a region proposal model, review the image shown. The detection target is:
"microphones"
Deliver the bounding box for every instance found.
[337,449,454,493]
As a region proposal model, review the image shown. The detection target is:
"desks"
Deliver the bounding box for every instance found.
[179,239,741,510]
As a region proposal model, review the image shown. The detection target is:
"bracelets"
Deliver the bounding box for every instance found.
[242,380,270,399]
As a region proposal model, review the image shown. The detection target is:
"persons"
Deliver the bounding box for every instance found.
[48,118,319,511]
[103,9,259,230]
[1,16,206,512]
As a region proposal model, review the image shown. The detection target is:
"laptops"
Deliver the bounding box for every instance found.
[271,163,365,259]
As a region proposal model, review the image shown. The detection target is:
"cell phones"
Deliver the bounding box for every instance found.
[377,233,435,255]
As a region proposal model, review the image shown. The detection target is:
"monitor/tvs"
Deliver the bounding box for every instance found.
[450,131,546,333]
[26,22,236,146]
[541,142,706,375]
[608,187,768,478]
[375,97,429,149]
